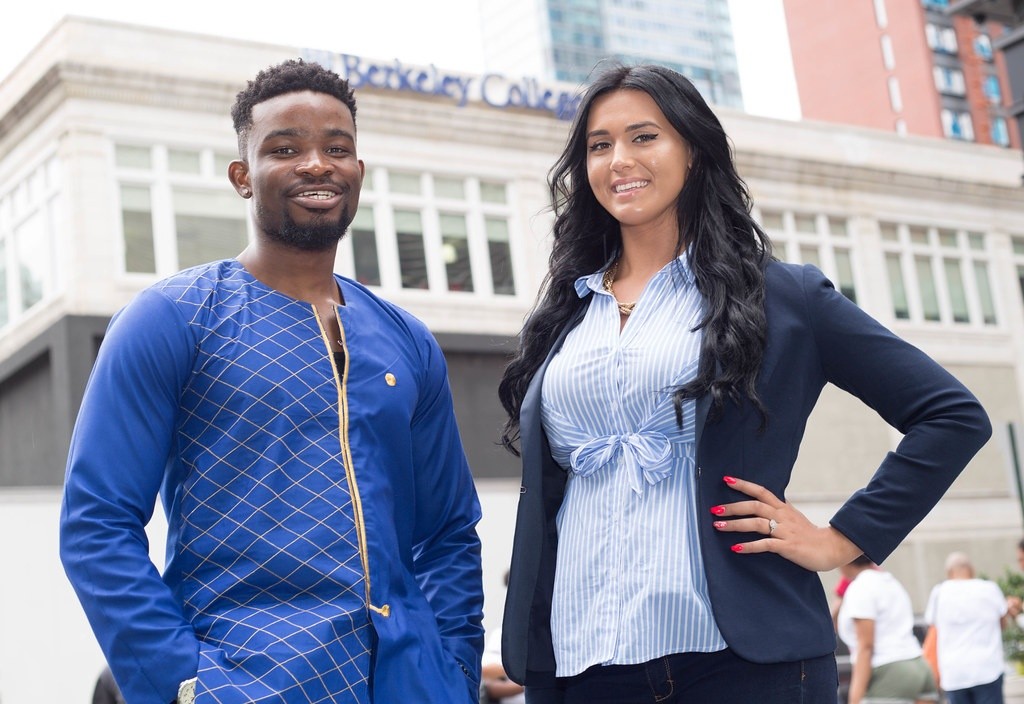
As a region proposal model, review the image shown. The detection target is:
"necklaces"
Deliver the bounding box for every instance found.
[602,257,642,318]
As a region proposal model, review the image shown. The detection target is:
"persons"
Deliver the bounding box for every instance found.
[494,58,992,704]
[830,548,938,704]
[1000,539,1024,649]
[480,661,525,704]
[923,553,1011,703]
[57,57,491,703]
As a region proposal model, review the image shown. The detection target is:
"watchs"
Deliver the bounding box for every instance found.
[177,675,196,704]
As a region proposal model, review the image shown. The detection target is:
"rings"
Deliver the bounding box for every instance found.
[768,518,777,536]
